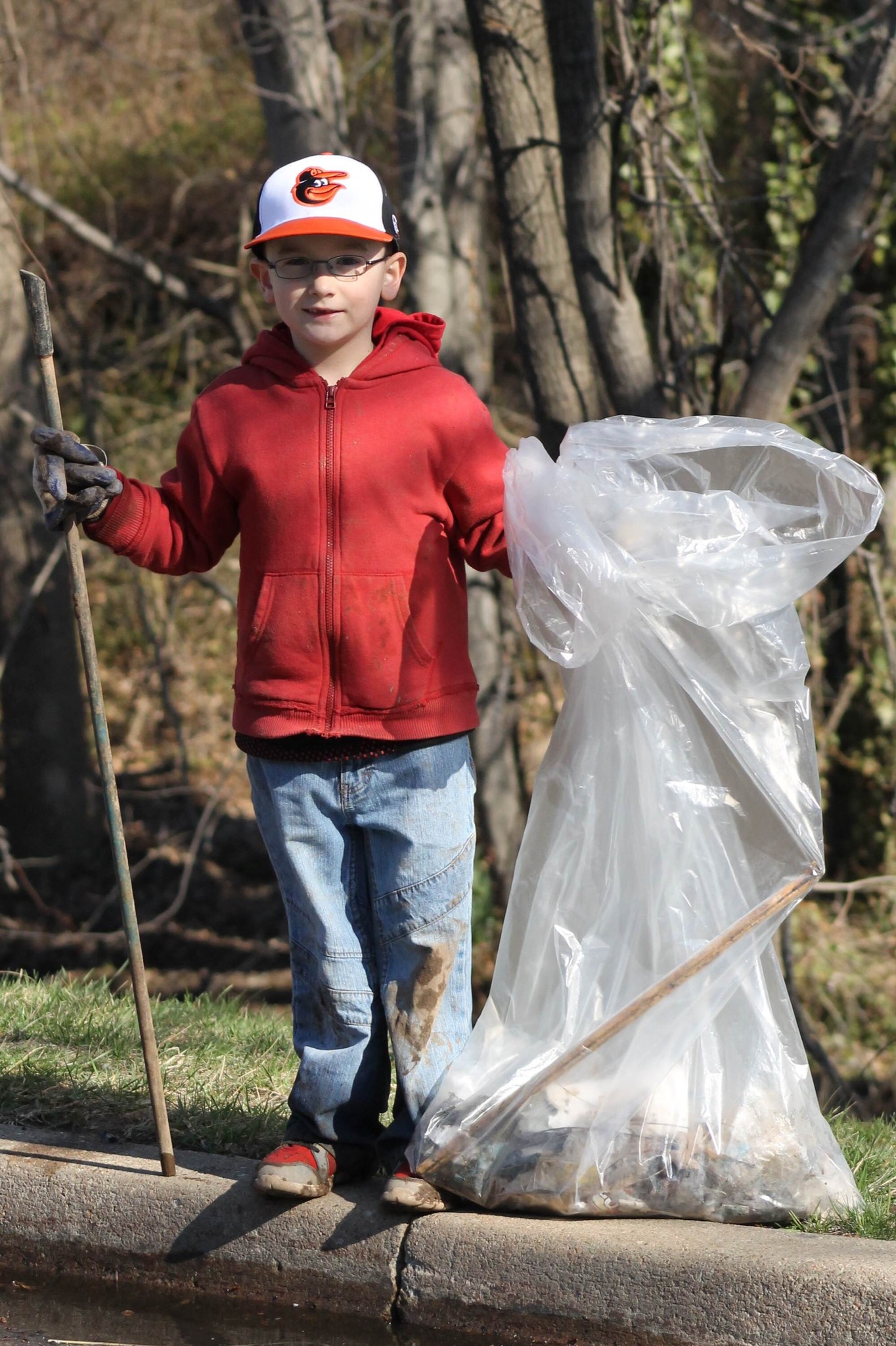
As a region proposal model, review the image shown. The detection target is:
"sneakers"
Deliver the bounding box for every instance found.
[383,1158,456,1212]
[254,1132,374,1198]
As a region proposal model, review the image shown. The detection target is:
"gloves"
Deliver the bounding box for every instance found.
[29,428,121,531]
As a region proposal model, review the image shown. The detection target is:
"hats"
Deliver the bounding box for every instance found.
[246,154,402,256]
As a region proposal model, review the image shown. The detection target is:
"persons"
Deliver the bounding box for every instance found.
[32,151,514,1214]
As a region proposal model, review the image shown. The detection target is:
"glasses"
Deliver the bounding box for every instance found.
[256,247,386,279]
